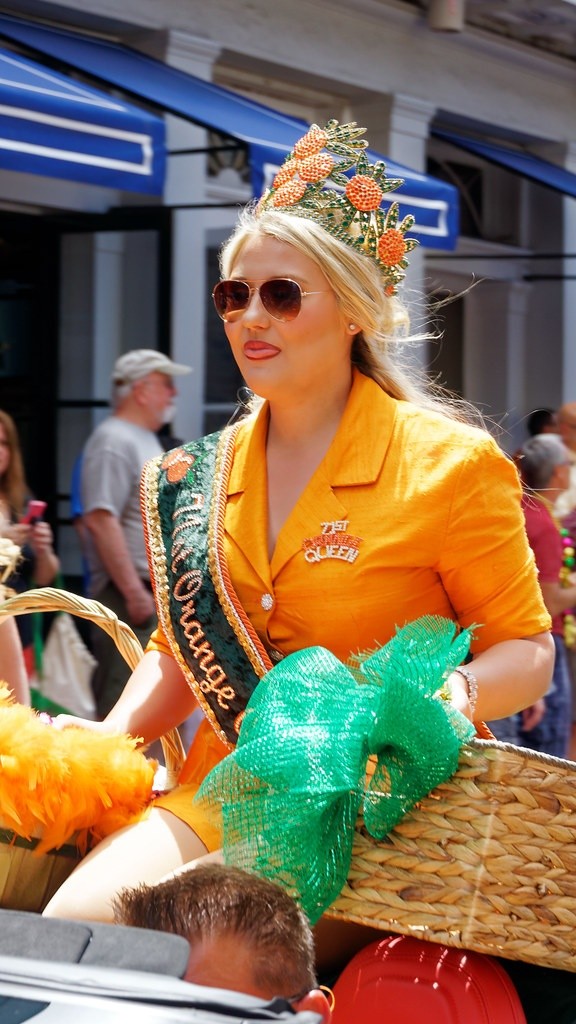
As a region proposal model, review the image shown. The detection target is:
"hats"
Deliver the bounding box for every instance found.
[107,349,196,394]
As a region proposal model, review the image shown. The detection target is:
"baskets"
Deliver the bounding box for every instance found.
[256,737,576,973]
[1,589,187,913]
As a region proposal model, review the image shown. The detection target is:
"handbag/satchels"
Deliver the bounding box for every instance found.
[31,612,96,722]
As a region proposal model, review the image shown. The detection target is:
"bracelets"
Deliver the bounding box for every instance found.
[454,664,477,715]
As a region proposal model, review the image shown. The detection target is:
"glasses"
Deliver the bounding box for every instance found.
[212,277,334,324]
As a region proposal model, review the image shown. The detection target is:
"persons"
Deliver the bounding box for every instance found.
[82,348,195,718]
[110,864,332,1023]
[1,411,59,709]
[527,406,558,438]
[44,120,556,1023]
[552,402,576,518]
[486,433,576,759]
[67,448,91,598]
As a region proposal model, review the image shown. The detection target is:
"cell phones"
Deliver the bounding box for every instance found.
[20,500,47,526]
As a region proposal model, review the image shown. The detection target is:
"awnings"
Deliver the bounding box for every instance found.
[0,19,459,252]
[0,48,166,196]
[429,125,576,198]
